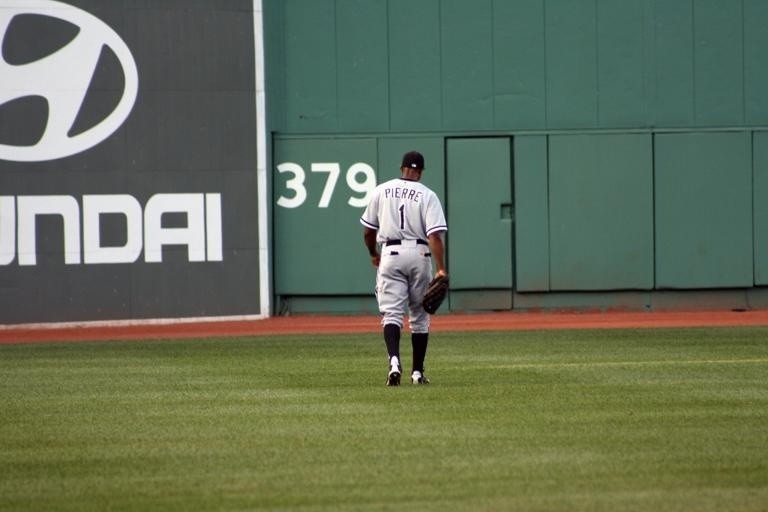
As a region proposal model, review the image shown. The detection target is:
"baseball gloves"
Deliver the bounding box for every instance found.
[422,275,448,315]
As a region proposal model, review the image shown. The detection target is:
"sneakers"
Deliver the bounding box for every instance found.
[410,370,432,386]
[385,363,403,387]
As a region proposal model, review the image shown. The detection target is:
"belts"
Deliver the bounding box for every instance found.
[381,239,429,248]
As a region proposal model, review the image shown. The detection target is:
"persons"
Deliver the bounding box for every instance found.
[360,151,449,387]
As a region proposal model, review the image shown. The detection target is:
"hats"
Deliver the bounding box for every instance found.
[400,151,426,170]
